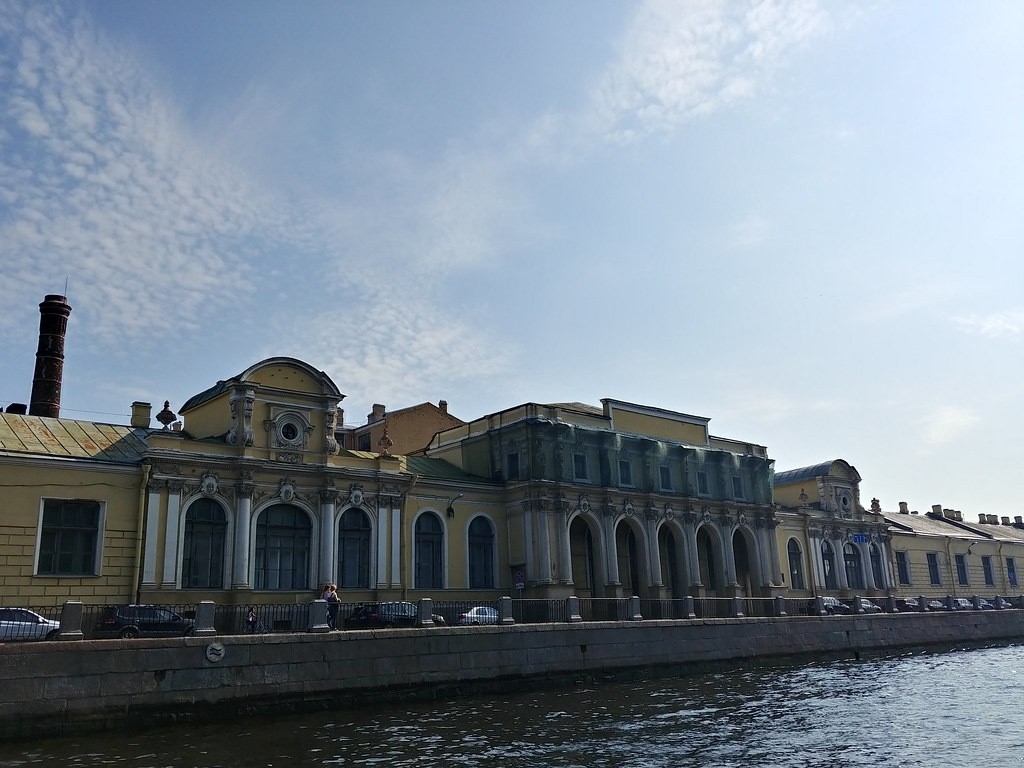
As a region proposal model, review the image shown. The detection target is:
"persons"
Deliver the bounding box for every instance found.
[245,609,258,633]
[320,585,332,627]
[327,585,341,631]
